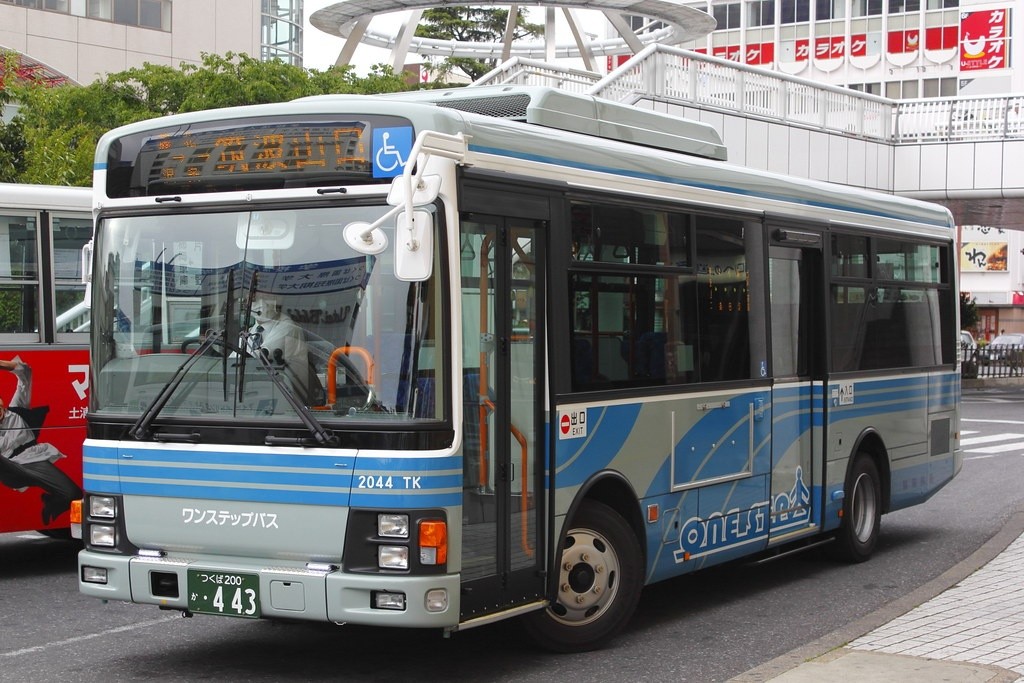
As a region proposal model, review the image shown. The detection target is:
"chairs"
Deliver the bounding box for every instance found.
[405,375,497,458]
[629,301,934,383]
[571,338,597,386]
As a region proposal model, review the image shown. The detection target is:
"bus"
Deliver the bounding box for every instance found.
[70,85,960,655]
[1,183,203,546]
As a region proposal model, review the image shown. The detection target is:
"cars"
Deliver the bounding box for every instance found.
[960,331,980,379]
[979,333,1024,365]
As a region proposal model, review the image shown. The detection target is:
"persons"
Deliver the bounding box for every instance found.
[227,268,309,402]
[0,355,82,525]
